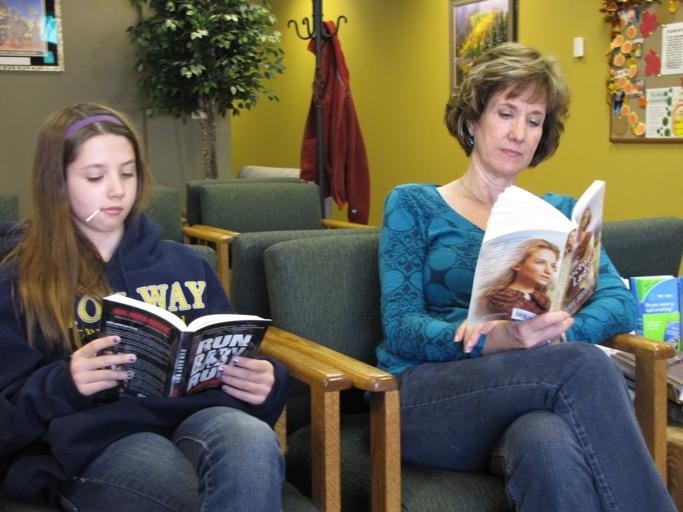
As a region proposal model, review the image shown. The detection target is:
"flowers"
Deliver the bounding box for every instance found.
[599,1,663,137]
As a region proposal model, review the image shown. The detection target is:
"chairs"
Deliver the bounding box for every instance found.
[187,244,353,510]
[178,176,379,325]
[138,182,185,247]
[595,217,681,510]
[2,193,21,224]
[258,232,512,511]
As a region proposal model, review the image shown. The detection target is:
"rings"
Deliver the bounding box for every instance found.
[544,337,552,345]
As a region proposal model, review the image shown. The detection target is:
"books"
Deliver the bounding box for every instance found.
[459,176,606,357]
[98,289,273,401]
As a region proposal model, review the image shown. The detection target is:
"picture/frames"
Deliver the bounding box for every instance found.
[607,0,683,143]
[0,0,65,71]
[449,0,517,104]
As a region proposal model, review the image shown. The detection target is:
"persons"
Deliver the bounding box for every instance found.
[562,209,596,309]
[361,39,683,512]
[476,239,560,320]
[0,98,292,510]
